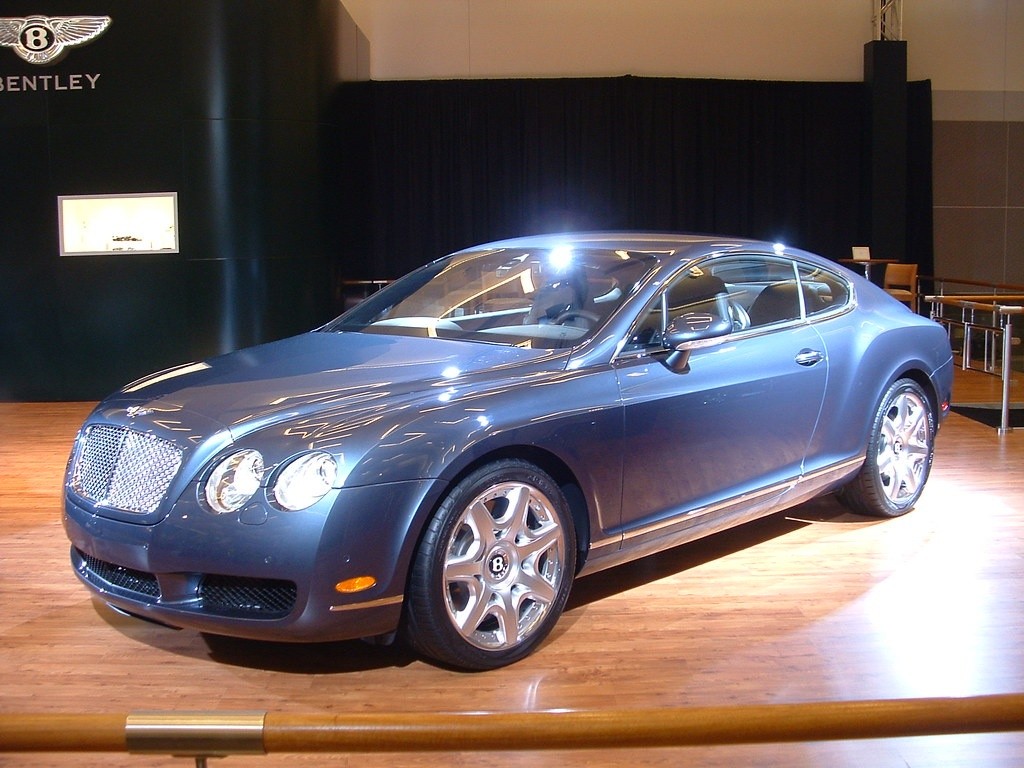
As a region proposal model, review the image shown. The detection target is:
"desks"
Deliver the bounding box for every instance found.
[345,281,395,298]
[840,258,899,281]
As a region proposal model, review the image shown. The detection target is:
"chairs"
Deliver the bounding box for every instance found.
[481,269,532,313]
[629,275,826,339]
[442,271,481,318]
[880,263,917,314]
[526,265,592,328]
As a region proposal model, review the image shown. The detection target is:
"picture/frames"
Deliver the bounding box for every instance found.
[58,192,179,256]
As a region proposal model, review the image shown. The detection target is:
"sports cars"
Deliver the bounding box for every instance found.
[60,230,955,670]
